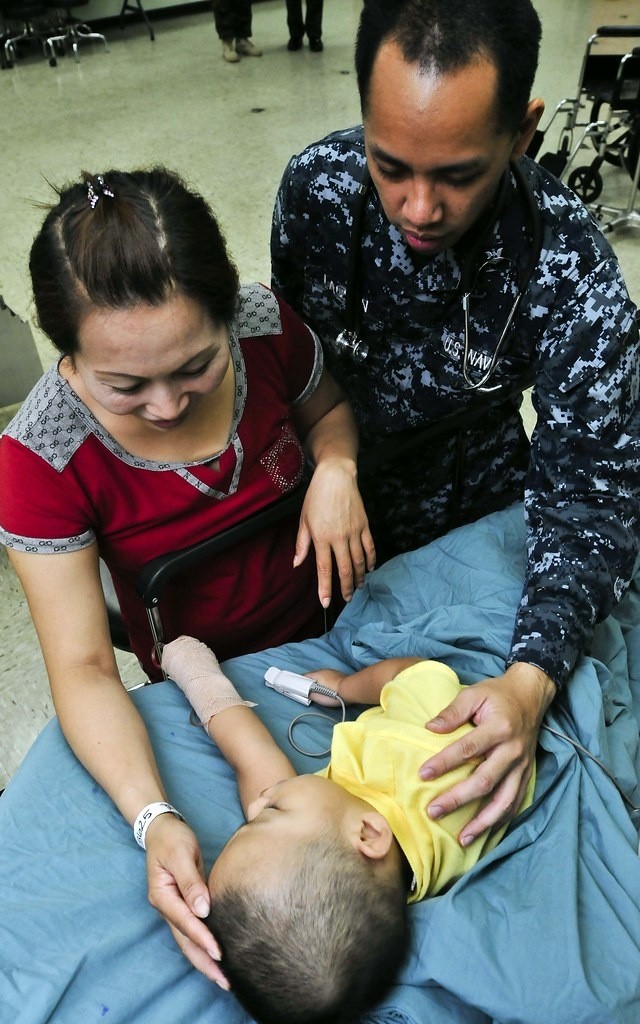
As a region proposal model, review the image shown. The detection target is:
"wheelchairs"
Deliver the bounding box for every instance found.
[525,20,640,242]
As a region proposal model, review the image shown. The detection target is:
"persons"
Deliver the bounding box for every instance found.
[214,0,327,62]
[151,636,534,1024]
[0,162,376,993]
[270,1,640,847]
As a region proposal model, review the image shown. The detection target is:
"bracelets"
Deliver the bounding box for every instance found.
[133,801,184,852]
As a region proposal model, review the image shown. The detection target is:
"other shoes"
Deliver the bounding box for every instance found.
[288,26,305,50]
[236,38,263,56]
[307,30,323,51]
[222,39,239,61]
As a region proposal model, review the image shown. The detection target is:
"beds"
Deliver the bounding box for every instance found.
[0,508,640,1022]
